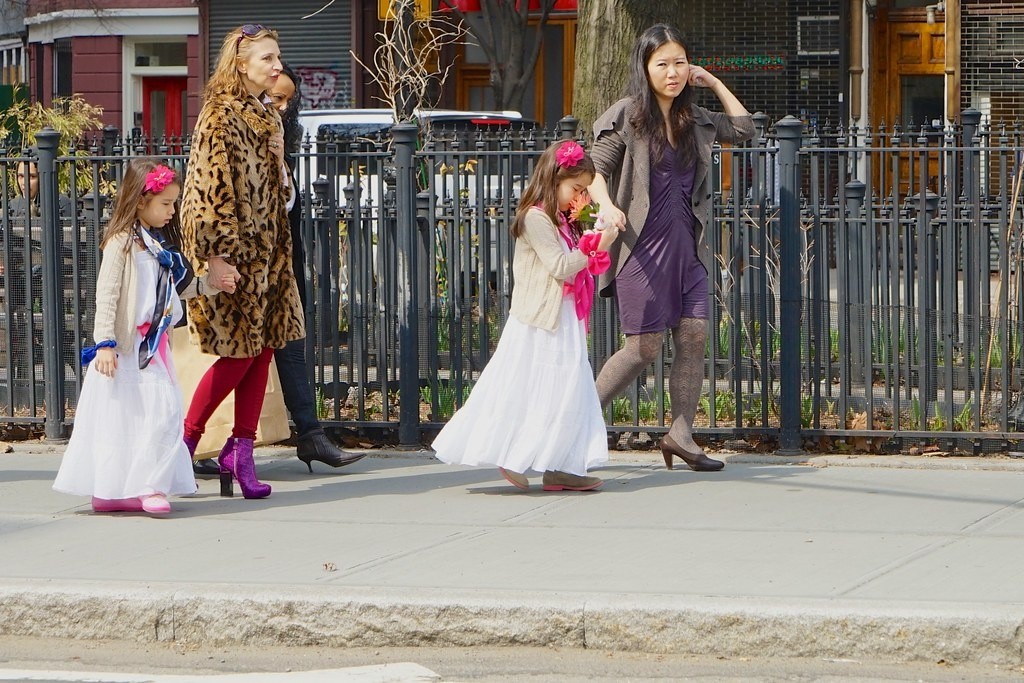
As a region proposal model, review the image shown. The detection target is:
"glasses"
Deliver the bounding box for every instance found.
[236,24,272,55]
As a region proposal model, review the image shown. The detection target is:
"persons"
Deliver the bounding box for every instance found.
[52,158,236,513]
[431,24,756,491]
[0,161,80,440]
[178,24,367,498]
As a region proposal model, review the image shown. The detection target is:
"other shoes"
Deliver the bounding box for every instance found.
[91,494,144,512]
[543,468,603,491]
[138,492,170,513]
[499,466,528,489]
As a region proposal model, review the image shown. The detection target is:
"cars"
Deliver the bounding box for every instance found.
[292,108,544,307]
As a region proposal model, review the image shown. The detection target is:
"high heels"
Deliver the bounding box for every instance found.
[660,434,724,471]
[192,457,219,474]
[218,437,271,499]
[296,429,366,472]
